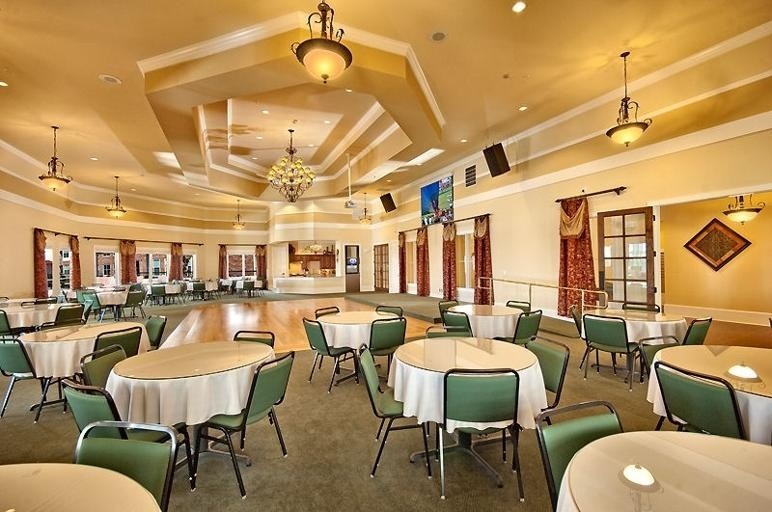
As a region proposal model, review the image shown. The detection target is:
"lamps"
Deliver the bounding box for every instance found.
[36,125,74,190]
[104,175,127,219]
[232,199,246,230]
[291,0,353,84]
[607,49,651,146]
[720,192,765,224]
[266,128,316,204]
[359,192,371,225]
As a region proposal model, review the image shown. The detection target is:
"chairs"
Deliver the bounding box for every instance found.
[302,299,771,512]
[1,277,295,512]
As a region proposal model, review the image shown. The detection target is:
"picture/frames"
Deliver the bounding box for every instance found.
[684,218,752,272]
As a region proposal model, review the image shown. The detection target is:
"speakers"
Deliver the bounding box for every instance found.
[483,143,511,178]
[379,193,397,214]
